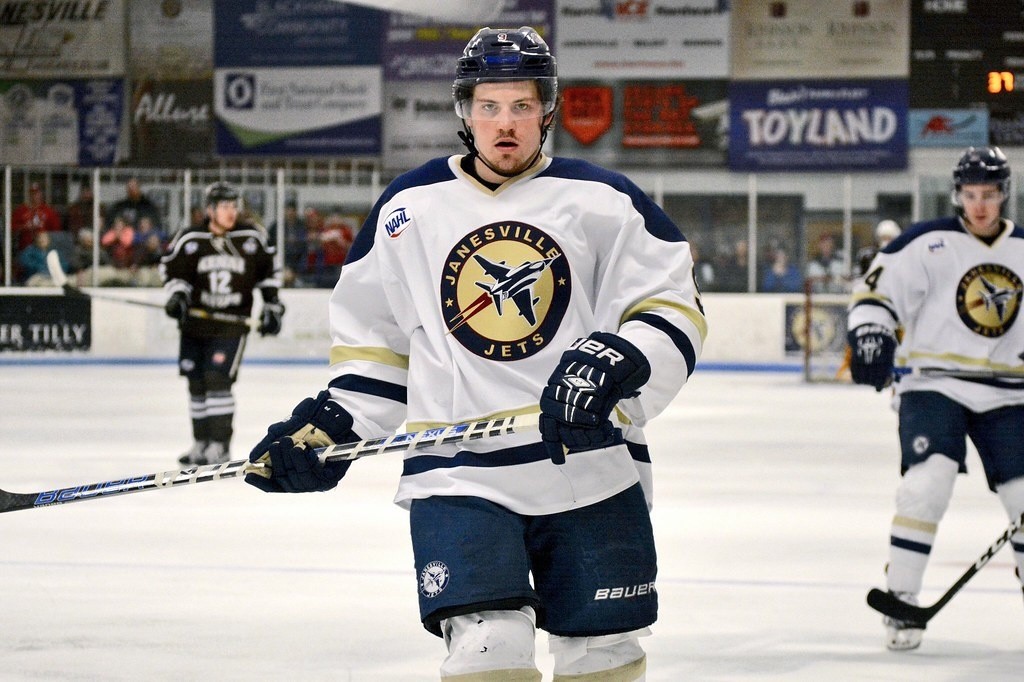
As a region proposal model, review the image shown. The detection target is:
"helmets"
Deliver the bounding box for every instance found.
[952,146,1012,197]
[876,219,901,241]
[201,181,242,213]
[452,27,557,119]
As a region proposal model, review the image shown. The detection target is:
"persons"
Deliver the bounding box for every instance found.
[846,147,1024,650]
[248,26,709,682]
[687,221,903,295]
[13,180,354,287]
[160,183,286,466]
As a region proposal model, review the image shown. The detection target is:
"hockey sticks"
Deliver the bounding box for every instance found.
[46,248,264,327]
[0,411,544,515]
[889,365,1024,380]
[866,509,1024,624]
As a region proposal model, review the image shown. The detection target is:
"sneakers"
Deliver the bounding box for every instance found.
[882,565,925,651]
[180,438,230,465]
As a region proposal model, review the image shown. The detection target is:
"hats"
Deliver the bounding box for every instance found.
[30,181,41,192]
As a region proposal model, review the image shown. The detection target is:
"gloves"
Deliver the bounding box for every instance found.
[847,323,898,393]
[539,330,649,466]
[165,289,189,320]
[259,304,283,335]
[240,390,362,493]
